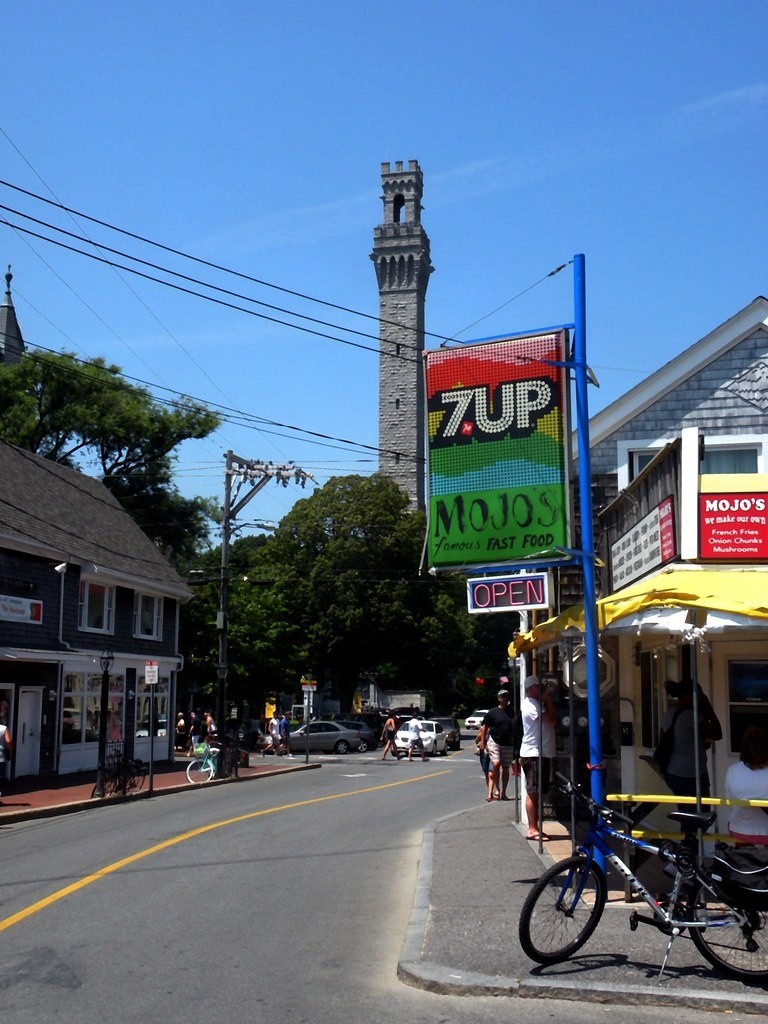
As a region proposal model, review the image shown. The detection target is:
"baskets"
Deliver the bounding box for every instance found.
[193,743,208,758]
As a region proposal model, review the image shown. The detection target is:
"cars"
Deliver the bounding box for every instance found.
[137,707,460,749]
[264,721,361,756]
[465,710,490,730]
[390,720,448,757]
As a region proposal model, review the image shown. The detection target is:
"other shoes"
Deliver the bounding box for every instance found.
[261,749,294,758]
[487,793,493,802]
[494,793,500,800]
[423,758,429,762]
[398,756,403,760]
[502,795,508,800]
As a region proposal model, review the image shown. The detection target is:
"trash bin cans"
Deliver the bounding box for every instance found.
[239,749,248,768]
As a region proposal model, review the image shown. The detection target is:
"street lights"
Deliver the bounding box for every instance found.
[221,524,274,770]
[95,643,115,799]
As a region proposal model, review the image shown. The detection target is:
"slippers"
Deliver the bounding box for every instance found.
[526,830,550,841]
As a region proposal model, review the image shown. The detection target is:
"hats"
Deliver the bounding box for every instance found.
[498,689,509,696]
[524,675,547,689]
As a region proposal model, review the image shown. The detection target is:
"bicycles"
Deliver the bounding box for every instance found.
[90,749,146,800]
[519,772,768,983]
[187,729,220,783]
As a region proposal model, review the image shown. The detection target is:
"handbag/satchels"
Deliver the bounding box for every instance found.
[386,719,395,736]
[1,748,10,761]
[475,741,481,755]
[207,717,217,732]
[710,839,768,911]
[177,729,185,735]
[657,703,694,764]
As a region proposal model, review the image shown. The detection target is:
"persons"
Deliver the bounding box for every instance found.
[476,690,514,802]
[380,712,404,760]
[519,675,557,840]
[726,727,768,848]
[653,679,711,847]
[261,711,293,757]
[408,712,429,762]
[176,711,215,757]
[0,715,13,796]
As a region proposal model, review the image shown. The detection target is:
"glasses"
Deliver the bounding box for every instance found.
[501,693,509,698]
